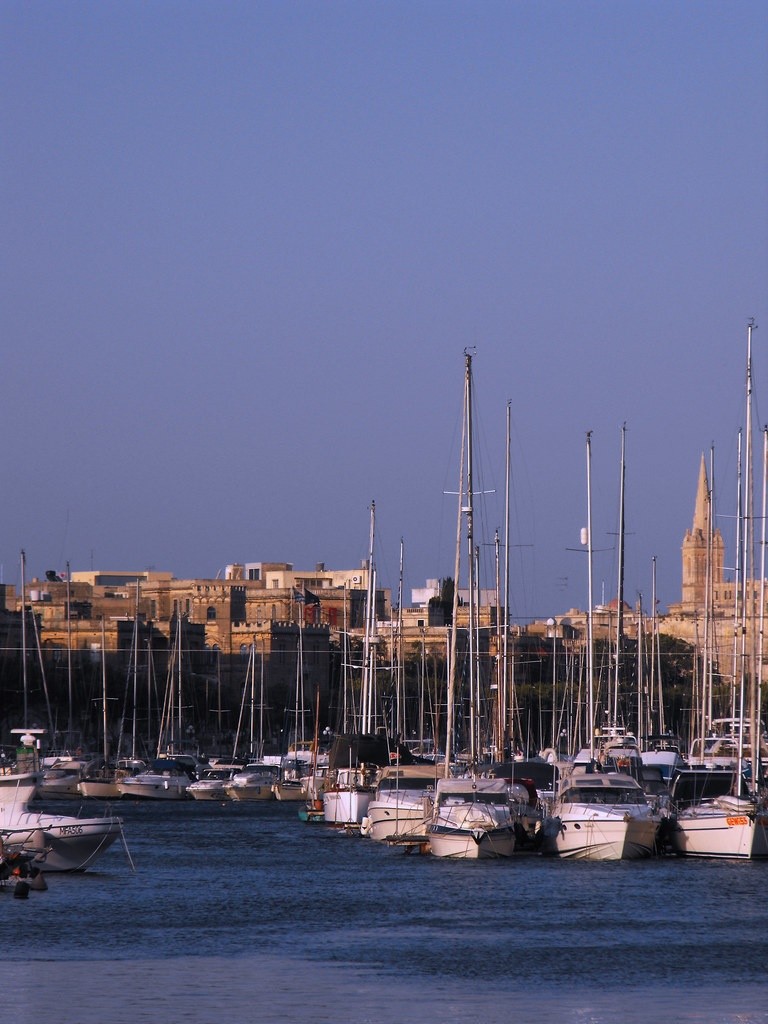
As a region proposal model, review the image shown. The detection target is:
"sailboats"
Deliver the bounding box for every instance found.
[673,319,768,861]
[540,421,678,864]
[0,549,124,874]
[16,345,555,858]
